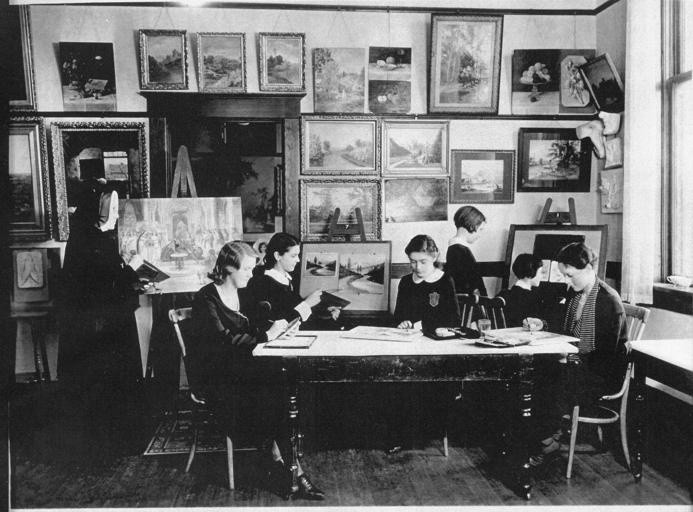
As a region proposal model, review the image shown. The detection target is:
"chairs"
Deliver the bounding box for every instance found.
[166,306,284,490]
[472,287,507,331]
[551,301,651,480]
[453,291,478,327]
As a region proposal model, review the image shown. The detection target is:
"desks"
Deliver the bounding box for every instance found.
[248,323,582,501]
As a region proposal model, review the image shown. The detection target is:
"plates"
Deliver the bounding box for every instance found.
[473,338,531,349]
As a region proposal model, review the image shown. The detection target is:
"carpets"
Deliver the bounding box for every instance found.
[140,408,262,457]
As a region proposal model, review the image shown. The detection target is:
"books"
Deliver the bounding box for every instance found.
[132,261,169,292]
[304,290,352,320]
[339,325,424,343]
[263,335,317,349]
[529,330,580,346]
[275,316,301,339]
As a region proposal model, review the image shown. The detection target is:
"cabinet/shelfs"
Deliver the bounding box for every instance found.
[629,337,693,494]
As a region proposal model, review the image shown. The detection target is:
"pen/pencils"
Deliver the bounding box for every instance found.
[407,326,408,332]
[269,320,274,323]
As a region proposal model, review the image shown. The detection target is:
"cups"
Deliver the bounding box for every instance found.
[478,318,491,339]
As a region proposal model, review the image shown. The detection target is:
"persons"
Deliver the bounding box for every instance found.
[192,240,327,499]
[56,186,154,461]
[532,242,629,471]
[446,206,495,331]
[503,255,550,332]
[256,232,342,330]
[383,235,461,454]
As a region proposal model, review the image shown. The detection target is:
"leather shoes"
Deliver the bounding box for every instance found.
[293,467,325,499]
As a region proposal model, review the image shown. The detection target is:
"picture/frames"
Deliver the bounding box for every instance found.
[120,196,243,293]
[384,180,448,224]
[381,120,449,176]
[427,13,500,114]
[450,150,515,204]
[315,48,365,112]
[7,116,54,244]
[301,113,380,175]
[7,245,63,312]
[2,1,37,112]
[137,30,188,91]
[260,32,306,92]
[518,127,595,194]
[301,244,390,313]
[504,224,609,295]
[57,42,118,111]
[51,120,147,241]
[513,50,624,116]
[368,47,412,116]
[298,178,383,243]
[196,32,248,94]
